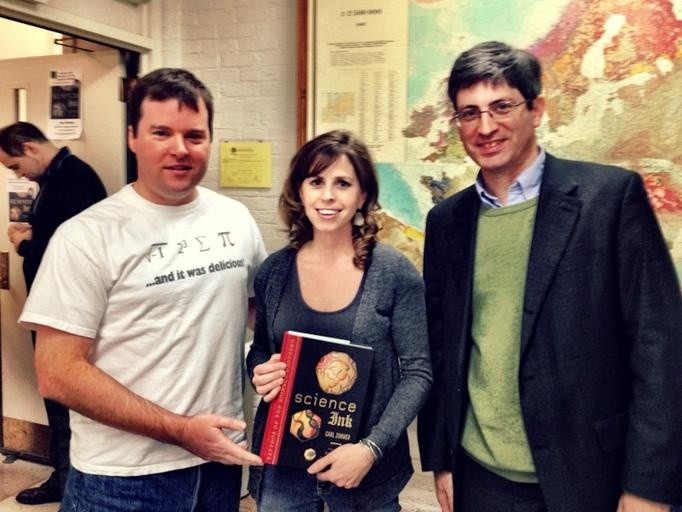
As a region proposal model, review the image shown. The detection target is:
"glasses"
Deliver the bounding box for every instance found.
[452,100,528,120]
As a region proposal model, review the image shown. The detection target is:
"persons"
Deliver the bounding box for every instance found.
[245,130,435,512]
[0,120,108,504]
[16,68,272,511]
[412,40,682,512]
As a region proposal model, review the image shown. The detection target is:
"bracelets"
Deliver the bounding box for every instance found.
[361,436,385,460]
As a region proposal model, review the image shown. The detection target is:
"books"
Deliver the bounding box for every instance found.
[260,329,375,476]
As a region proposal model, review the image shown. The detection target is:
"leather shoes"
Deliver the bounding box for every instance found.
[16,479,63,504]
